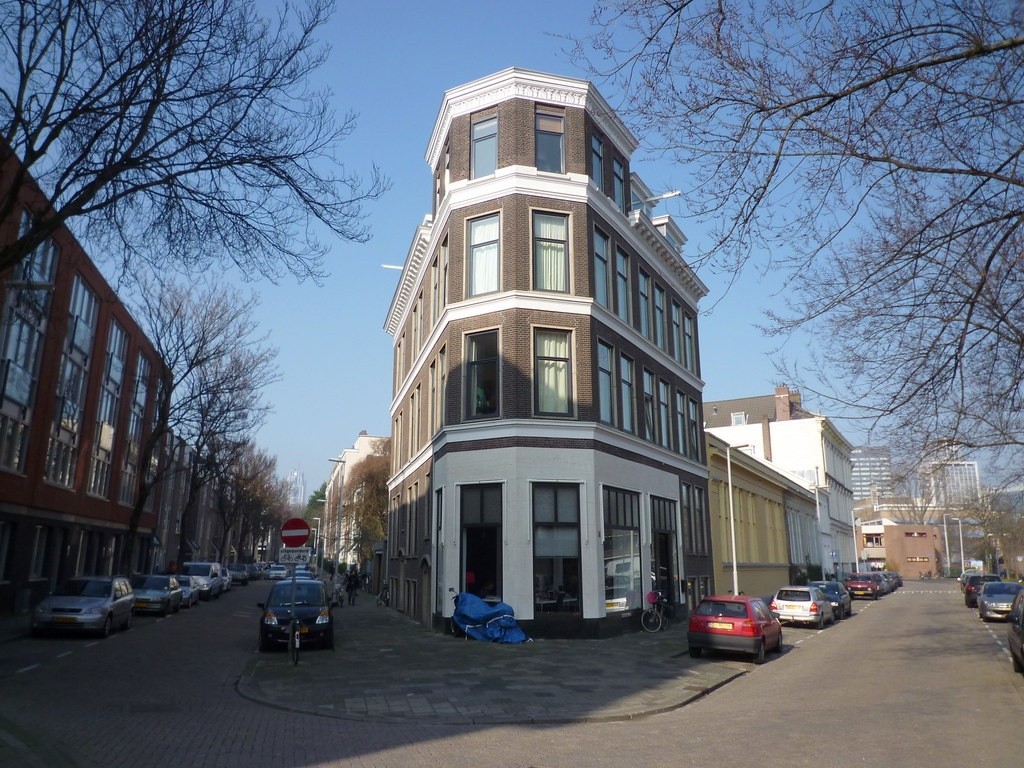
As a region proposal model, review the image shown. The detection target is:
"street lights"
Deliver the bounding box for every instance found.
[950,517,964,576]
[942,513,954,579]
[331,457,348,609]
[310,498,328,571]
[850,506,865,574]
[727,444,752,593]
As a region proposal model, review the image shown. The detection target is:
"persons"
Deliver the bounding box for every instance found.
[304,562,335,581]
[346,570,359,606]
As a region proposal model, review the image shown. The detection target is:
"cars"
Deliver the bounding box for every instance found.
[1004,589,1024,675]
[805,580,850,619]
[217,562,311,593]
[965,573,1002,609]
[688,594,783,661]
[256,579,334,653]
[181,561,220,600]
[170,574,200,605]
[771,586,832,626]
[127,575,182,614]
[34,575,135,635]
[841,571,902,600]
[604,586,630,613]
[977,582,1024,621]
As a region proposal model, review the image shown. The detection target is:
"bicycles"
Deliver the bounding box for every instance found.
[280,598,305,664]
[638,588,675,634]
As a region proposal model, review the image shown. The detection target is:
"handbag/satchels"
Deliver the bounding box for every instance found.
[355,588,360,597]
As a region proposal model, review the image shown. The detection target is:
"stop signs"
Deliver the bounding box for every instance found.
[281,518,310,547]
